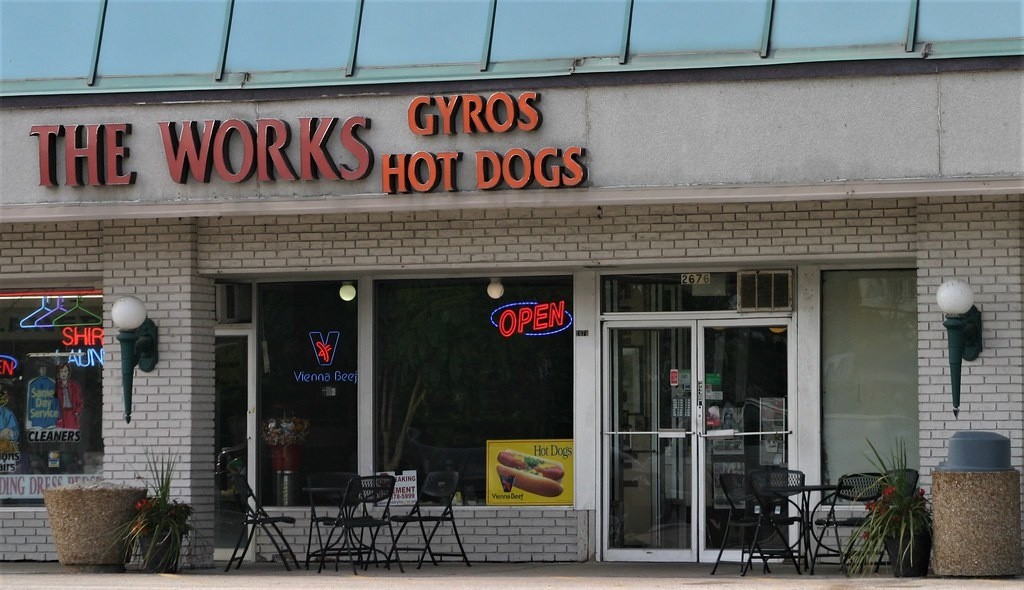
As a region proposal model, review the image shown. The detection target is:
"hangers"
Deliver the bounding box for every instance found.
[53,297,102,327]
[20,298,52,328]
[34,296,67,327]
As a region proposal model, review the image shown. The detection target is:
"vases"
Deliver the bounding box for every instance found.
[881,528,932,577]
[139,531,183,574]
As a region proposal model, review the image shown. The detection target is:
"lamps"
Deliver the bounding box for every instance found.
[936,279,983,421]
[486,278,504,299]
[111,295,159,424]
[339,281,356,301]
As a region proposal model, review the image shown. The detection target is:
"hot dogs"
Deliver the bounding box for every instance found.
[496,449,565,497]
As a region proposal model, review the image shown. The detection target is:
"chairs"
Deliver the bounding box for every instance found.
[865,469,919,573]
[808,473,890,576]
[749,469,810,570]
[710,474,803,576]
[223,468,471,576]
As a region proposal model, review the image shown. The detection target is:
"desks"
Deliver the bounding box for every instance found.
[301,486,391,571]
[761,485,854,571]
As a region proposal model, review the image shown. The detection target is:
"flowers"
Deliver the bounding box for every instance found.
[98,442,213,573]
[840,435,933,578]
